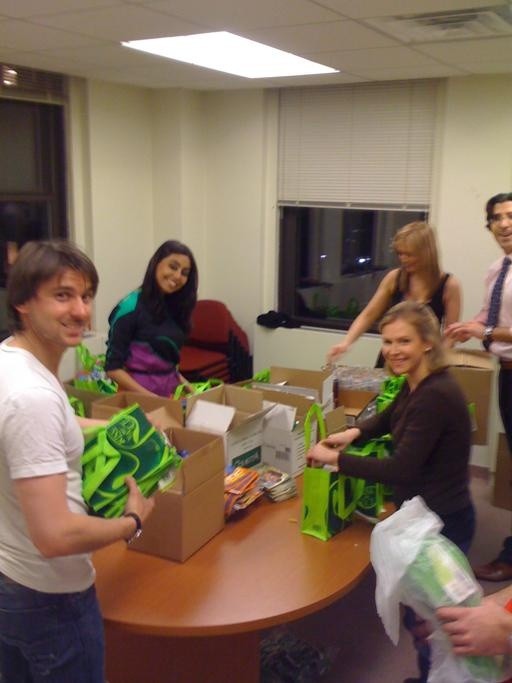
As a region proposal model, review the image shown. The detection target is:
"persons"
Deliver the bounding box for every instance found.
[306,302,476,682]
[0,241,147,683]
[324,221,464,369]
[444,192,512,582]
[104,239,199,401]
[436,599,512,661]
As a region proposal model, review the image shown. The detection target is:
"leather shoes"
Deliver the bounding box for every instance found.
[474,559,512,581]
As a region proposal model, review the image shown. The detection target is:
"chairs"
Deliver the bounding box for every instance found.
[178,300,252,389]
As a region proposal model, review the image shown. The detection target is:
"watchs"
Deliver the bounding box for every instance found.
[483,327,495,337]
[123,512,143,545]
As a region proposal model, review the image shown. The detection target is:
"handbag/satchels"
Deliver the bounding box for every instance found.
[299,372,406,541]
[78,347,183,520]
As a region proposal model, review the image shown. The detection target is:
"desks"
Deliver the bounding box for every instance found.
[90,469,394,681]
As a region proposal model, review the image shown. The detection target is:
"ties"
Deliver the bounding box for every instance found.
[483,258,511,352]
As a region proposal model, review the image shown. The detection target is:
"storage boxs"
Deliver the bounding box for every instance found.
[185,385,278,522]
[261,387,319,502]
[488,434,512,510]
[92,391,184,435]
[126,428,223,561]
[303,402,358,542]
[353,439,389,521]
[334,387,380,427]
[253,364,337,416]
[438,350,495,446]
